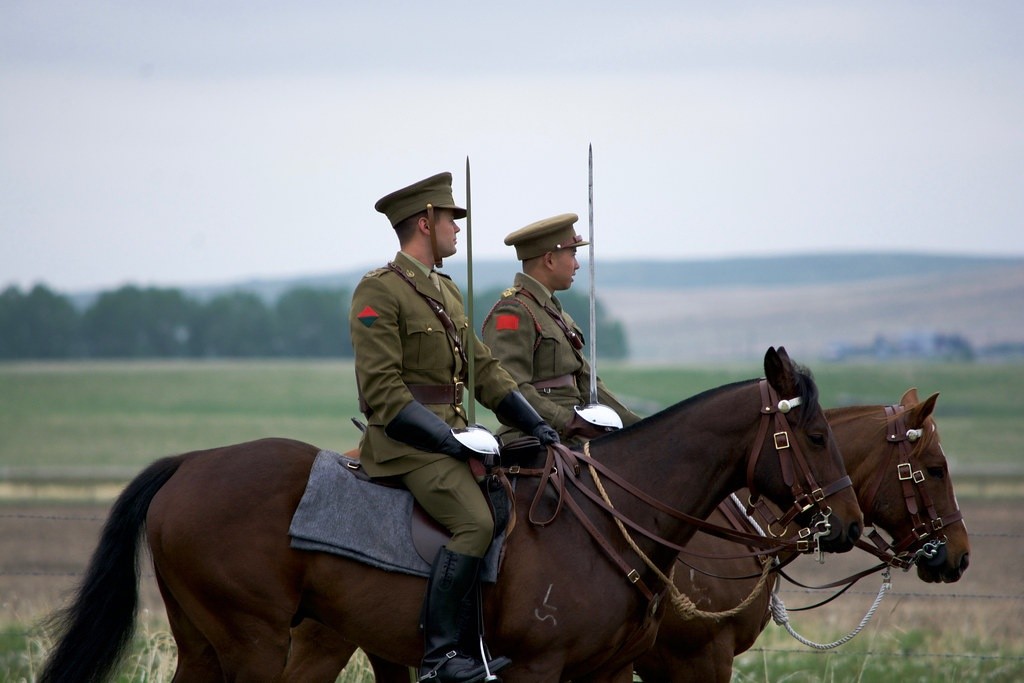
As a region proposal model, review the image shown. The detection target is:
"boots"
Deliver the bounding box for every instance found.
[417,545,512,683]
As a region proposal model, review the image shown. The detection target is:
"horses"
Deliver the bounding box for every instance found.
[20,345,864,683]
[279,386,970,683]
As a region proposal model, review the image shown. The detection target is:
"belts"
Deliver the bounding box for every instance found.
[359,381,464,412]
[531,373,579,390]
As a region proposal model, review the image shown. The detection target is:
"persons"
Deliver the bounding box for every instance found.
[483,211,644,450]
[349,172,562,683]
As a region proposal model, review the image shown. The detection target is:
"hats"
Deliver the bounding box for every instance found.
[504,213,589,260]
[374,171,467,228]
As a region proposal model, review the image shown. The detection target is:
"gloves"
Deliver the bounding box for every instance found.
[384,399,473,463]
[564,411,608,437]
[494,389,561,445]
